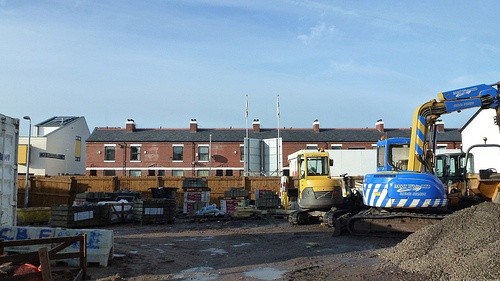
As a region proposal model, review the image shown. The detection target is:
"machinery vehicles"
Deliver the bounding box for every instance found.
[346,80,500,236]
[287,148,354,231]
[397,151,500,212]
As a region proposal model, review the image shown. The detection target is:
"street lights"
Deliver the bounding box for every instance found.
[23,115,32,208]
